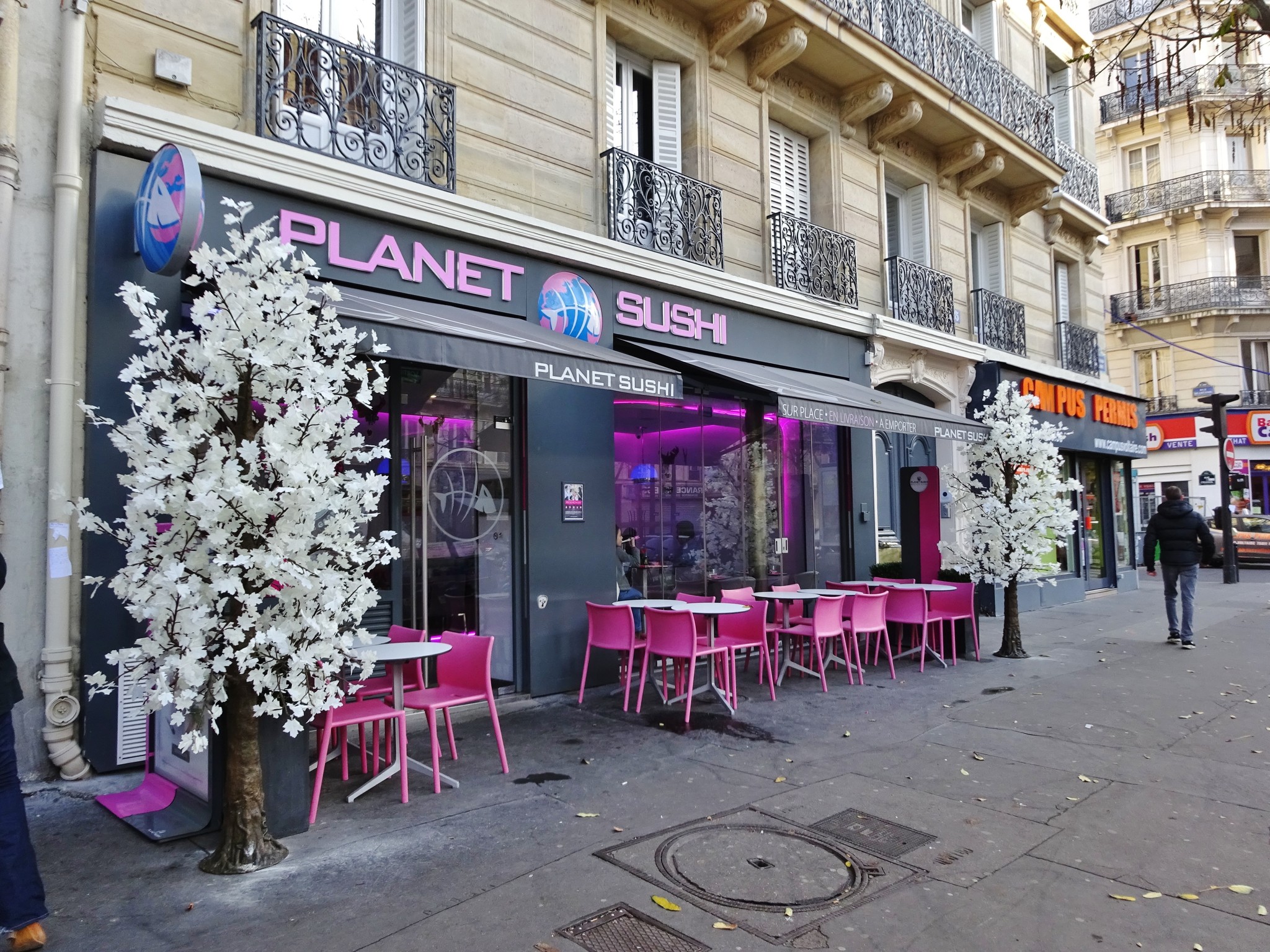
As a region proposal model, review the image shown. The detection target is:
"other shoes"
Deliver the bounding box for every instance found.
[11,922,46,952]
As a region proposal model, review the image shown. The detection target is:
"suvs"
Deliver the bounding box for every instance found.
[639,536,738,592]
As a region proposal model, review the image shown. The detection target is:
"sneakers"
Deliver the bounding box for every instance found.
[1167,633,1181,642]
[1182,640,1196,649]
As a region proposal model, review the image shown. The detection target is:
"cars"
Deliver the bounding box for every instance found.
[1081,521,1101,554]
[1201,514,1270,570]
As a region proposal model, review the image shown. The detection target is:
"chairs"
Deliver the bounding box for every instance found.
[579,572,981,724]
[309,624,510,821]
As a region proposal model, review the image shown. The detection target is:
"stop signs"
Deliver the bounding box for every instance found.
[1223,438,1235,472]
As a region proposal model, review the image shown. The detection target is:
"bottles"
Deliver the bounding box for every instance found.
[640,545,647,565]
[643,556,650,565]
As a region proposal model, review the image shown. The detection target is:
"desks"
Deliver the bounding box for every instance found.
[309,636,391,774]
[611,565,958,716]
[344,642,460,803]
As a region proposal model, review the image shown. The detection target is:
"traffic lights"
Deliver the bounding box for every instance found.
[1196,393,1240,440]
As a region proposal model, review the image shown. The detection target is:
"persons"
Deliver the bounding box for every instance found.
[1143,486,1215,649]
[616,525,648,639]
[670,521,703,581]
[0,621,50,952]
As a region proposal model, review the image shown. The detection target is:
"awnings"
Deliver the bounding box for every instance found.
[295,278,684,400]
[615,336,994,445]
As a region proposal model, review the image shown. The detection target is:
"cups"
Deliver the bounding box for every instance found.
[1116,532,1125,546]
[621,544,626,550]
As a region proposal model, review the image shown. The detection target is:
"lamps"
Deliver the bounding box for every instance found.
[629,427,658,486]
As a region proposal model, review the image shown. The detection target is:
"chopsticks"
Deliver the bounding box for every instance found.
[622,536,640,543]
